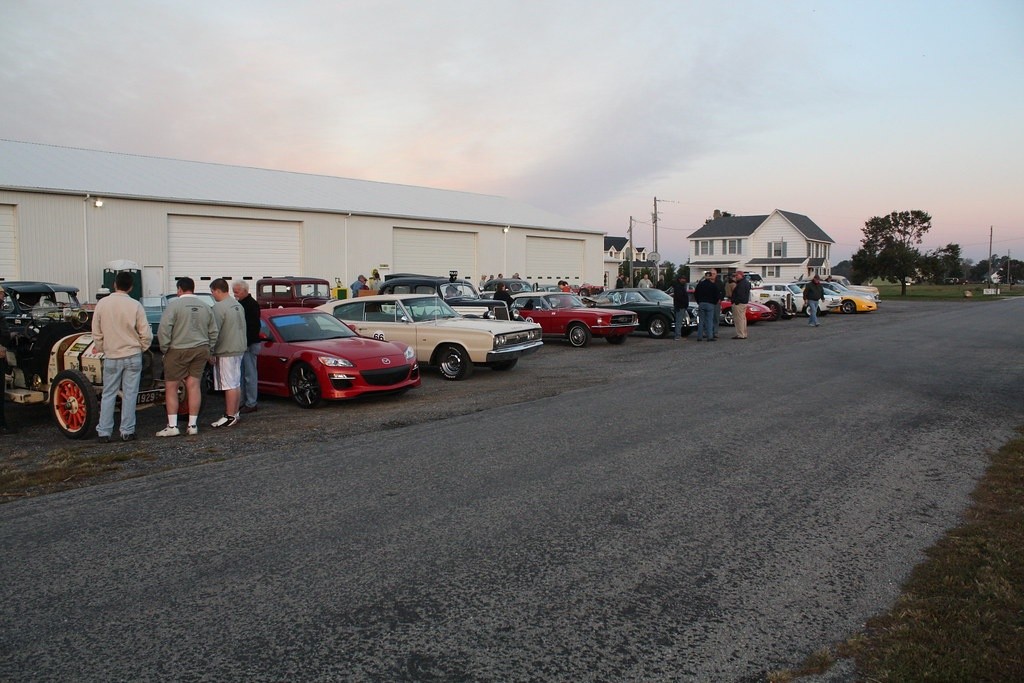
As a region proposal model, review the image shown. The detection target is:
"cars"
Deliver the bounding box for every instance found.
[0,276,422,440]
[592,288,699,339]
[378,271,640,347]
[313,294,543,380]
[665,275,882,327]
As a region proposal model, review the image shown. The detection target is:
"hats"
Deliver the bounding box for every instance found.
[828,275,832,278]
[732,271,744,275]
[814,274,820,280]
[678,275,687,279]
[705,272,712,277]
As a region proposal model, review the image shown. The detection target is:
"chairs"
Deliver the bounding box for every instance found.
[281,324,308,341]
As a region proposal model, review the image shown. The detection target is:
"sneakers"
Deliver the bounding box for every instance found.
[186,424,197,435]
[155,424,180,437]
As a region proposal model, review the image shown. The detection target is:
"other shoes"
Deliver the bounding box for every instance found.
[675,336,686,341]
[122,432,136,441]
[815,323,820,327]
[714,334,719,338]
[696,338,703,342]
[239,406,257,413]
[210,414,237,428]
[707,338,717,341]
[808,324,815,327]
[99,436,109,442]
[732,335,747,339]
[233,415,240,422]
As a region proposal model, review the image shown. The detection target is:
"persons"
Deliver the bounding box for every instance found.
[803,274,825,327]
[558,280,571,305]
[479,274,503,286]
[370,273,382,290]
[656,275,664,290]
[730,271,750,339]
[446,286,453,297]
[694,272,718,341]
[211,278,247,428]
[725,275,737,298]
[702,268,726,339]
[637,273,653,288]
[155,277,218,436]
[616,274,632,289]
[673,274,688,340]
[351,275,370,298]
[232,280,261,414]
[493,283,514,320]
[513,272,521,279]
[92,272,153,445]
